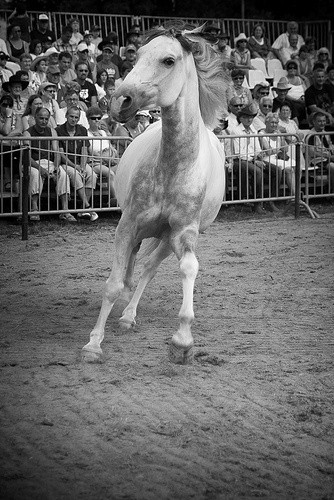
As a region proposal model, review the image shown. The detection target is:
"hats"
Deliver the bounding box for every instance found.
[103,44,113,52]
[0,46,60,92]
[237,105,258,124]
[217,33,248,45]
[125,44,137,52]
[91,25,101,31]
[76,43,89,52]
[284,60,298,71]
[253,77,292,98]
[39,14,48,20]
[83,32,94,38]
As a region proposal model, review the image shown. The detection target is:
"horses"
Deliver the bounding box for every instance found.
[82,22,237,364]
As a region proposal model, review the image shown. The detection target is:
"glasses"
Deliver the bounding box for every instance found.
[230,103,244,107]
[2,103,13,109]
[234,77,243,80]
[287,66,297,70]
[76,69,88,72]
[238,39,247,44]
[149,110,161,114]
[260,91,270,96]
[15,30,21,33]
[89,117,102,120]
[262,104,273,109]
[45,90,56,93]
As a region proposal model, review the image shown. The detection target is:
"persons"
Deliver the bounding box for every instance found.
[203,21,334,216]
[0,4,162,221]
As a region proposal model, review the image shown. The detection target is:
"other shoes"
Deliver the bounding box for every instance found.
[16,197,118,221]
[242,190,305,214]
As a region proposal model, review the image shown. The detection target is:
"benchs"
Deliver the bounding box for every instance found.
[0,172,329,198]
